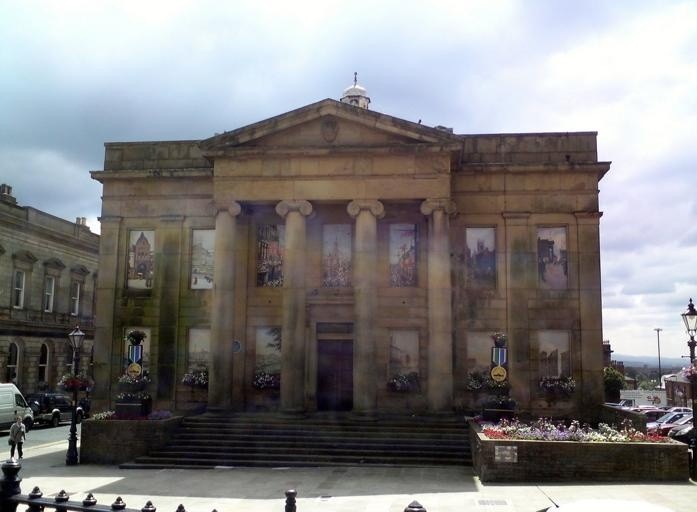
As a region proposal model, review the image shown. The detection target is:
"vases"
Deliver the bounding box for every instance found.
[131,338,141,345]
[118,384,146,393]
[190,383,208,389]
[65,385,86,392]
[114,398,153,417]
[495,339,503,348]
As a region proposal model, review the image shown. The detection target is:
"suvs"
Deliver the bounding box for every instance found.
[27,393,84,427]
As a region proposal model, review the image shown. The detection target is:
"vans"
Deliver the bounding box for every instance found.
[0,383,35,432]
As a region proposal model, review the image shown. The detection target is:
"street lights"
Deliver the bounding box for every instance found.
[680,298,697,481]
[654,328,663,390]
[66,326,85,467]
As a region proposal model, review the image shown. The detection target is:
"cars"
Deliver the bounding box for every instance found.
[604,398,697,448]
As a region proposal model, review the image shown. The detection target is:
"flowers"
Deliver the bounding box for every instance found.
[182,367,208,386]
[115,392,150,400]
[117,374,151,385]
[251,371,274,392]
[385,372,422,393]
[60,371,96,393]
[494,332,505,347]
[539,375,576,394]
[126,331,146,342]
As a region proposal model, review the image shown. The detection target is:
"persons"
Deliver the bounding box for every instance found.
[9,415,25,461]
